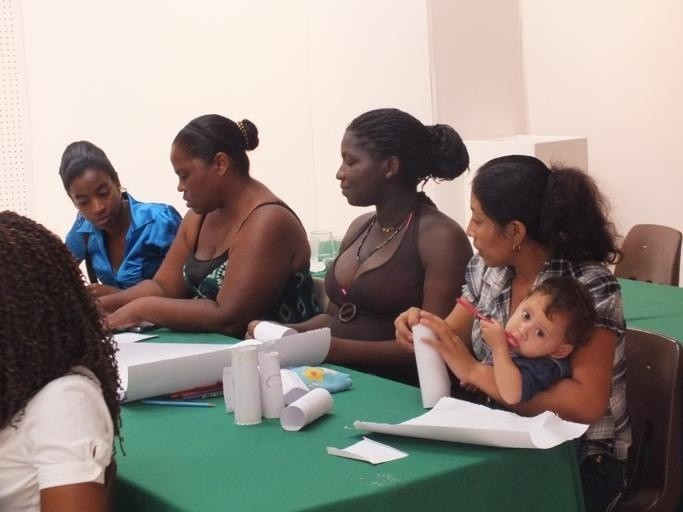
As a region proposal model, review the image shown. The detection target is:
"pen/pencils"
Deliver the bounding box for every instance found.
[457,298,513,338]
[141,383,223,407]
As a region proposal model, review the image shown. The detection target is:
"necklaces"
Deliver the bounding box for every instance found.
[373,223,397,233]
[356,215,408,265]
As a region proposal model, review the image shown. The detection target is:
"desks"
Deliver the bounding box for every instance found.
[615,278,682,340]
[113,329,585,509]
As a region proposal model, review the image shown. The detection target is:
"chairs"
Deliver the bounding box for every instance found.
[621,328,682,508]
[612,223,681,287]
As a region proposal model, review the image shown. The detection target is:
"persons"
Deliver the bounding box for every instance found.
[59,140,182,289]
[245,108,474,387]
[0,210,127,512]
[461,275,598,414]
[392,155,632,512]
[91,114,323,339]
